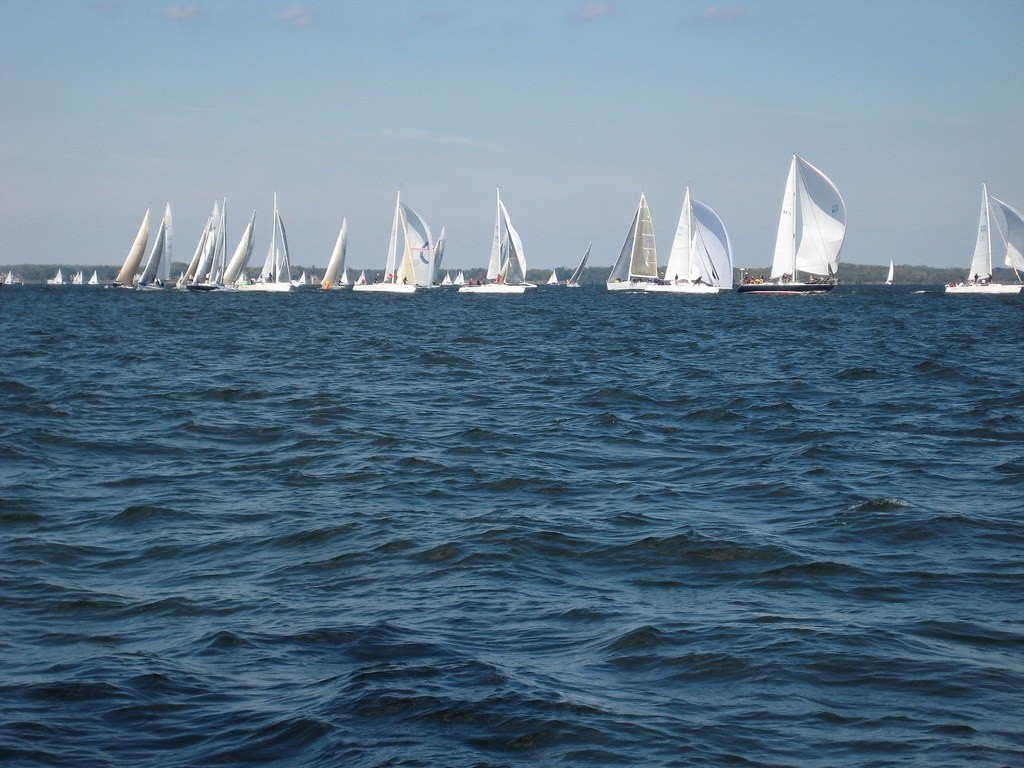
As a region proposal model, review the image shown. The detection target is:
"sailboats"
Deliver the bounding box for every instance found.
[353,190,437,293]
[440,186,538,293]
[736,153,847,295]
[945,183,1024,296]
[884,258,895,286]
[546,270,560,287]
[565,241,593,288]
[4,270,15,285]
[44,191,369,292]
[422,225,447,291]
[606,187,736,295]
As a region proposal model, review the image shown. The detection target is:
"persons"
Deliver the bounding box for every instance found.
[389,273,393,283]
[403,276,409,285]
[250,272,273,285]
[497,274,502,285]
[743,272,765,285]
[468,280,486,287]
